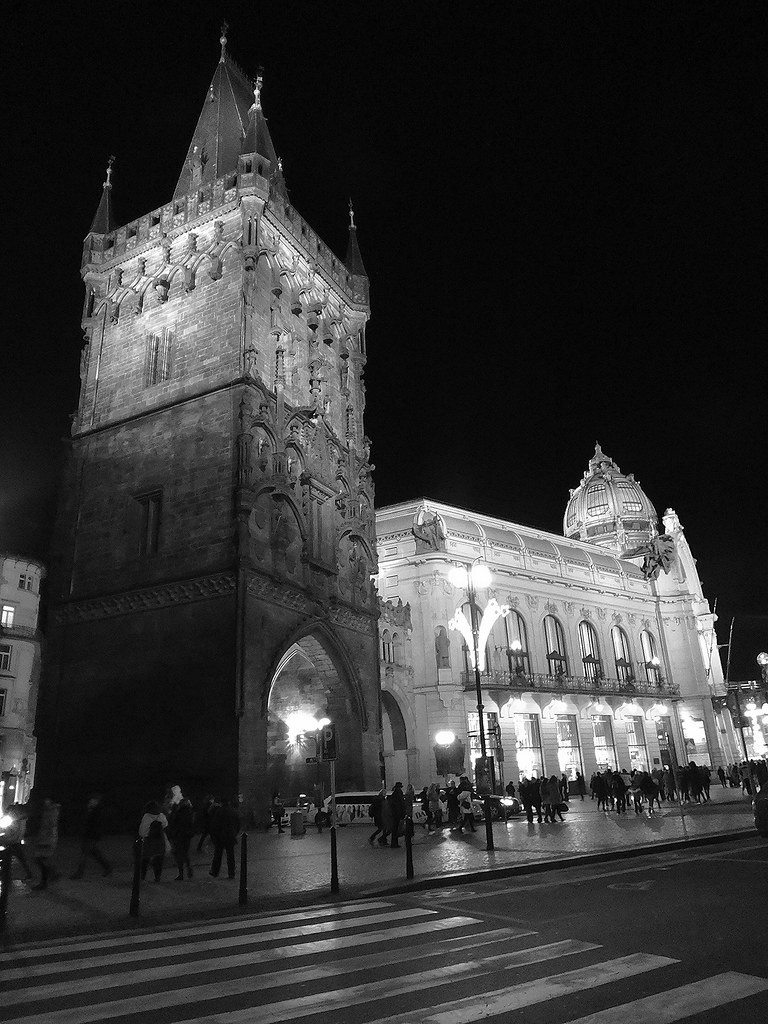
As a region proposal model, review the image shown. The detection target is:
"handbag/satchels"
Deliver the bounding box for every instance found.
[461,800,470,809]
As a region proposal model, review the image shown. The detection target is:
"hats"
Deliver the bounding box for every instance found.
[393,782,404,789]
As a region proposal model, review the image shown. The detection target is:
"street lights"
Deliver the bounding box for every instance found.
[435,726,454,787]
[448,561,498,854]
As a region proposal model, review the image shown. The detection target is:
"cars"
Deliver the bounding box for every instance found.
[302,790,522,827]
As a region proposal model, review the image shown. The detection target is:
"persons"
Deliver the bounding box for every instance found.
[30,795,62,892]
[368,789,391,846]
[0,805,33,890]
[272,797,285,834]
[403,783,415,836]
[167,785,183,815]
[138,800,168,882]
[378,782,405,849]
[416,757,768,836]
[204,796,241,880]
[68,794,112,882]
[197,794,216,853]
[165,798,197,881]
[204,797,225,850]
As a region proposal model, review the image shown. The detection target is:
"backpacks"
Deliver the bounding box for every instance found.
[149,816,165,845]
[368,802,377,818]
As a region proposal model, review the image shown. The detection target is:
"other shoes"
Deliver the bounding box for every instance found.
[153,868,163,882]
[421,822,478,837]
[209,871,219,877]
[229,873,234,879]
[140,871,146,880]
[21,872,34,883]
[368,836,402,849]
[264,826,268,832]
[68,870,85,880]
[102,866,114,877]
[591,796,711,814]
[48,874,59,882]
[186,872,192,878]
[174,874,183,880]
[527,816,565,823]
[31,883,47,891]
[278,829,285,833]
